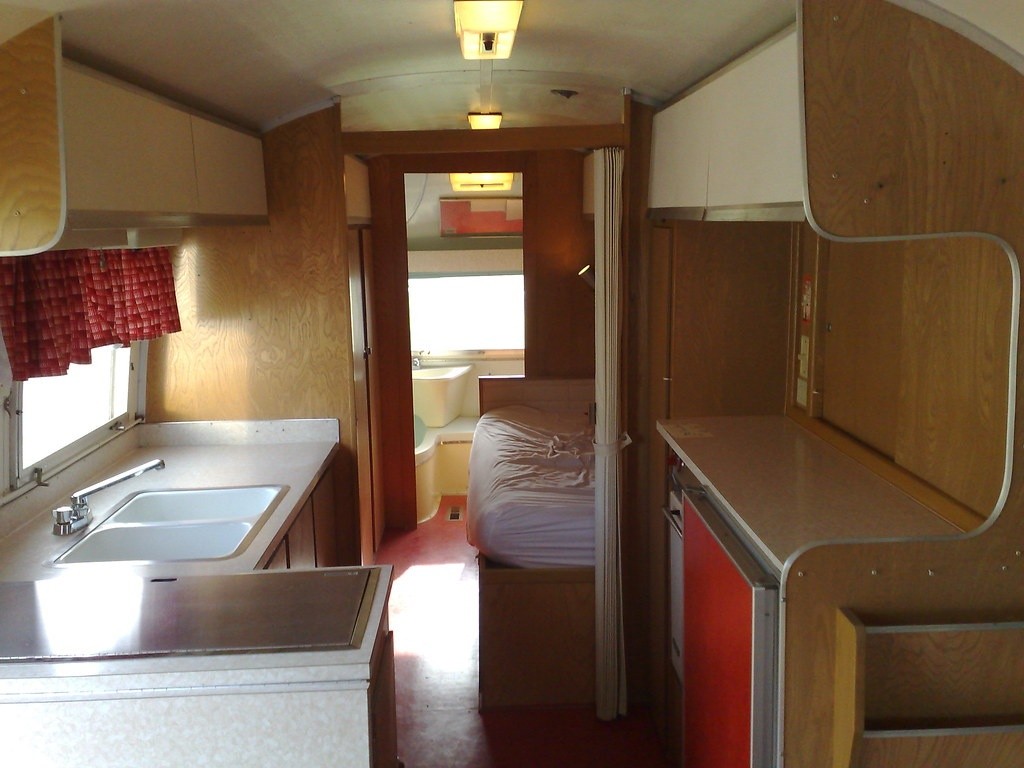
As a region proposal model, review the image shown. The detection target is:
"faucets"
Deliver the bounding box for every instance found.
[53,458,166,537]
[412,356,421,370]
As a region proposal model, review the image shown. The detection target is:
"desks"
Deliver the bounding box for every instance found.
[655,407,984,587]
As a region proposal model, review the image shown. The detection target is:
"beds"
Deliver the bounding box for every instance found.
[466,378,595,712]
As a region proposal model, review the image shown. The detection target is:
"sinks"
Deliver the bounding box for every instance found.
[54,522,264,562]
[111,484,292,522]
[412,358,474,428]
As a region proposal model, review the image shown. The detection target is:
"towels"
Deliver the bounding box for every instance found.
[1,246,182,380]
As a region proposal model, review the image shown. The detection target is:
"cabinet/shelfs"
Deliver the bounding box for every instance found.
[42,53,270,251]
[267,468,335,574]
[646,20,806,222]
